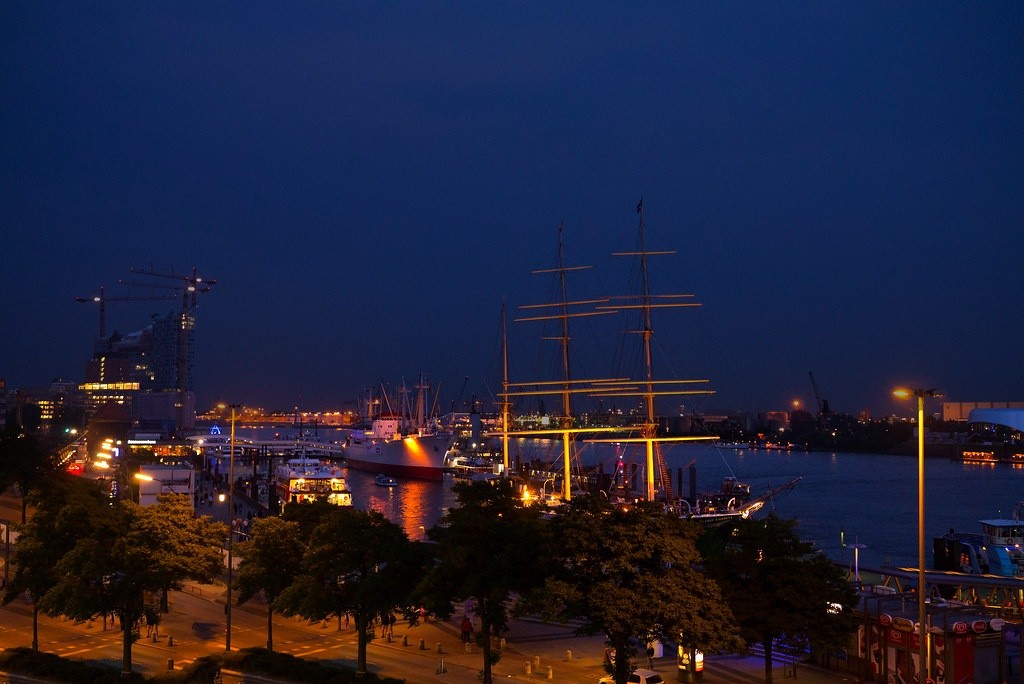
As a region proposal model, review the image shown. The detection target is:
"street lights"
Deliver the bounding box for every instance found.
[221,400,246,652]
[891,385,945,684]
[135,473,177,496]
[93,436,134,505]
[199,432,204,493]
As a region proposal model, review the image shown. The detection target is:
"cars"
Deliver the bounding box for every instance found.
[599,665,665,684]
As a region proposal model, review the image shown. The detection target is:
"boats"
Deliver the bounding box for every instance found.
[931,512,1024,578]
[341,370,456,482]
[710,441,804,450]
[374,472,399,488]
[442,411,504,483]
[237,441,355,515]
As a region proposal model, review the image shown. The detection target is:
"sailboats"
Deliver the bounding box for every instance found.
[480,196,763,528]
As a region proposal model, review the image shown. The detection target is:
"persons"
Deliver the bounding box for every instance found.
[145,613,160,638]
[109,612,114,631]
[343,613,349,630]
[461,614,473,643]
[232,508,263,541]
[421,606,428,622]
[380,610,396,638]
[964,594,1024,624]
[195,473,242,515]
[646,644,655,669]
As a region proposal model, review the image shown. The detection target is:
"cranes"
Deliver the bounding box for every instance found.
[128,261,218,313]
[117,278,211,390]
[73,286,178,336]
